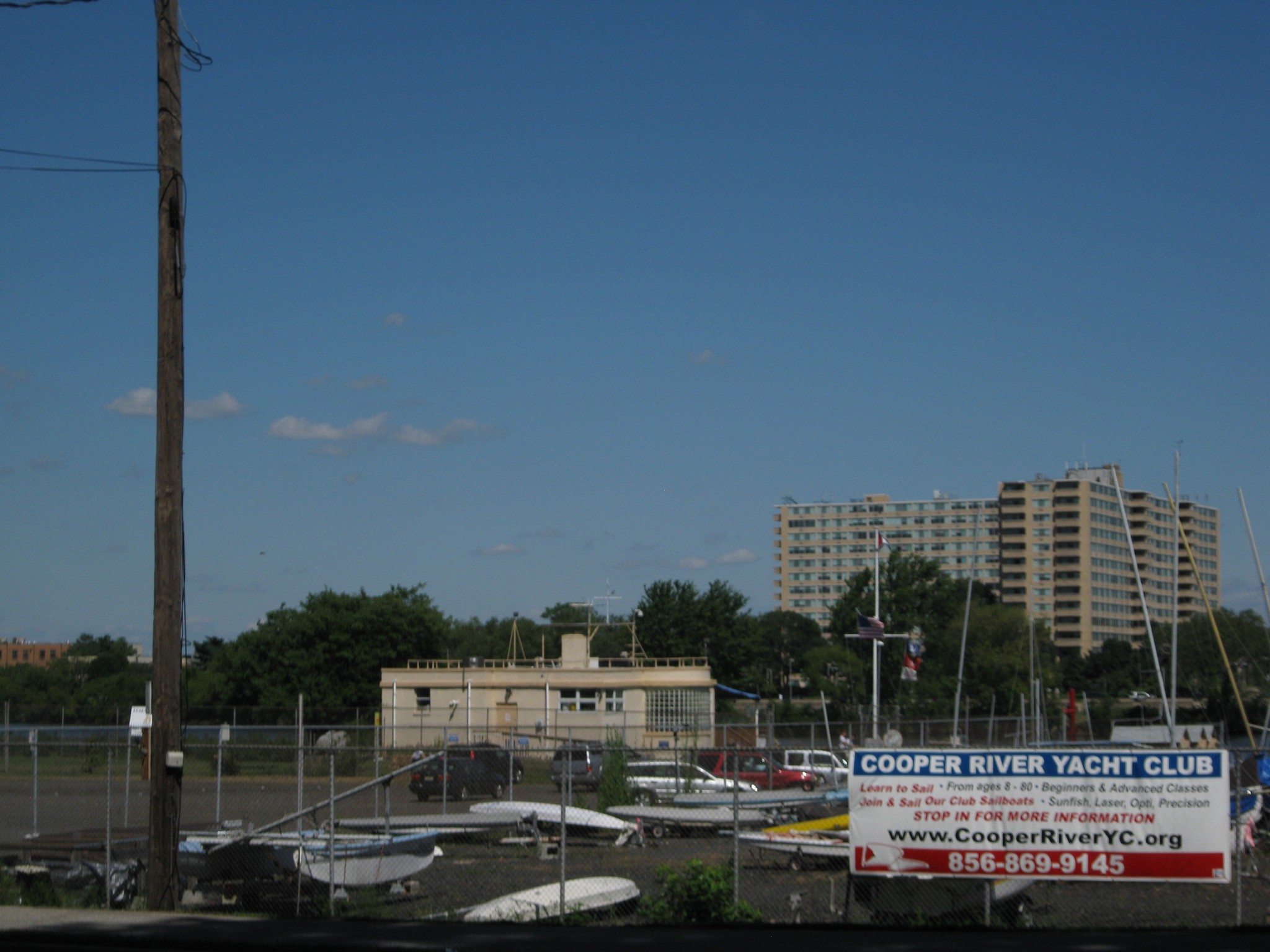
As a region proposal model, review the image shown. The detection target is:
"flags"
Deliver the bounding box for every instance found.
[857,615,885,638]
[900,626,926,684]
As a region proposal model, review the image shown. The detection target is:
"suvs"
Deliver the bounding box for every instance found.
[549,741,648,791]
[622,761,761,806]
[698,749,825,792]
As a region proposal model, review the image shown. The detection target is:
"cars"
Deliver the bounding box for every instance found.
[408,743,525,802]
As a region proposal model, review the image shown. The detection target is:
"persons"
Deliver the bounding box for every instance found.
[838,730,856,750]
[410,744,423,763]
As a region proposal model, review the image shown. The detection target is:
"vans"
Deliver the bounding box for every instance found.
[778,747,852,791]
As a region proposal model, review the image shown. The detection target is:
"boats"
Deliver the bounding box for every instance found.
[840,869,1038,916]
[735,827,853,869]
[603,801,772,831]
[669,788,831,810]
[1229,782,1264,854]
[462,875,641,926]
[469,801,642,838]
[332,810,539,839]
[181,827,440,894]
